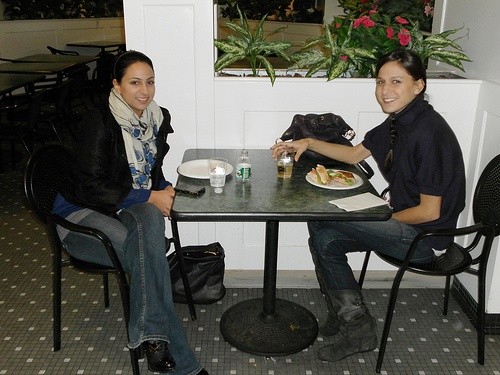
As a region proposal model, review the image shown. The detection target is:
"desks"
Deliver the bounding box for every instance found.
[0,63,74,74]
[73,40,125,51]
[14,54,100,64]
[172,148,391,356]
[0,74,47,95]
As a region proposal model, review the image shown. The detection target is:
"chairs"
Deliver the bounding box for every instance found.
[358,153,500,373]
[24,143,198,375]
[0,46,127,154]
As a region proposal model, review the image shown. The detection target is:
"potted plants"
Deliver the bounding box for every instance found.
[213,3,292,87]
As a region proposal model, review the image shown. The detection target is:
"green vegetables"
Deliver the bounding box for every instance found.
[318,168,354,185]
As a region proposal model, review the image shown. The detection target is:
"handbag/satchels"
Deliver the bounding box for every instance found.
[281,114,373,180]
[166,243,226,305]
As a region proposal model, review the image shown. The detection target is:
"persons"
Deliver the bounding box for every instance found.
[271,51,467,363]
[49,50,208,375]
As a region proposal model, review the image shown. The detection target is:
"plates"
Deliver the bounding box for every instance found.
[177,159,234,180]
[305,170,364,191]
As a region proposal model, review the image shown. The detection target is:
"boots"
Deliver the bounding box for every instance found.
[317,287,342,336]
[317,289,377,361]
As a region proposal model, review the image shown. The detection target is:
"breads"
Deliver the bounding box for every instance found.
[307,164,328,184]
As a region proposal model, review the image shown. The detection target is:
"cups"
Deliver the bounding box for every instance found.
[208,157,228,187]
[276,148,295,179]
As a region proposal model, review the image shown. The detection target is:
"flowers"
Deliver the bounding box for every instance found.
[287,0,475,81]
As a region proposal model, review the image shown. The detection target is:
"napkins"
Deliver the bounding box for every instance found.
[328,192,389,212]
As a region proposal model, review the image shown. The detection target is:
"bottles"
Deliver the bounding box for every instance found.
[235,148,252,183]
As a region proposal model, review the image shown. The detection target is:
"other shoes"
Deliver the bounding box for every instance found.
[196,368,209,375]
[137,340,176,371]
